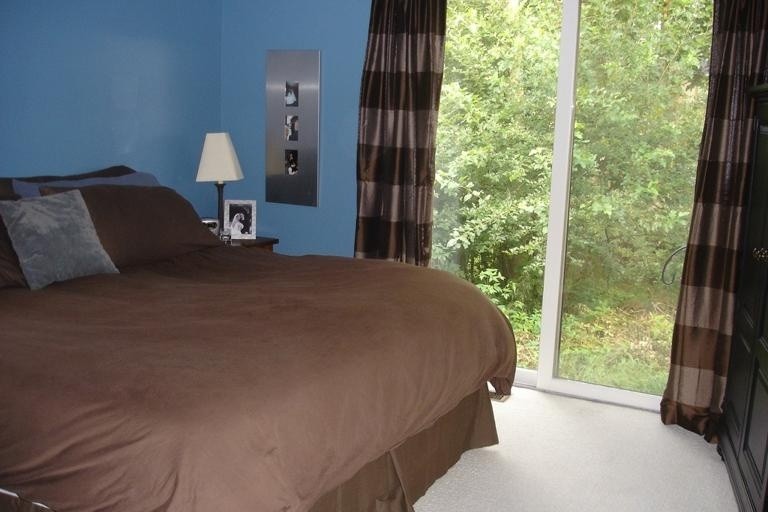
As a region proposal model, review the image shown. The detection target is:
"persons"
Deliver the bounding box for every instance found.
[232,214,244,235]
[239,211,251,234]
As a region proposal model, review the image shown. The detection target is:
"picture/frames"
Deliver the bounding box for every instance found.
[223,199,257,241]
[265,49,322,207]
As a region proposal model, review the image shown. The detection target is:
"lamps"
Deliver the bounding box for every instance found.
[196,132,244,237]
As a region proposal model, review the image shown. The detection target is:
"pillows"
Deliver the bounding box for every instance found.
[0,172,225,291]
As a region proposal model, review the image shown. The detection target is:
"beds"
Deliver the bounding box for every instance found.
[0,166,518,511]
